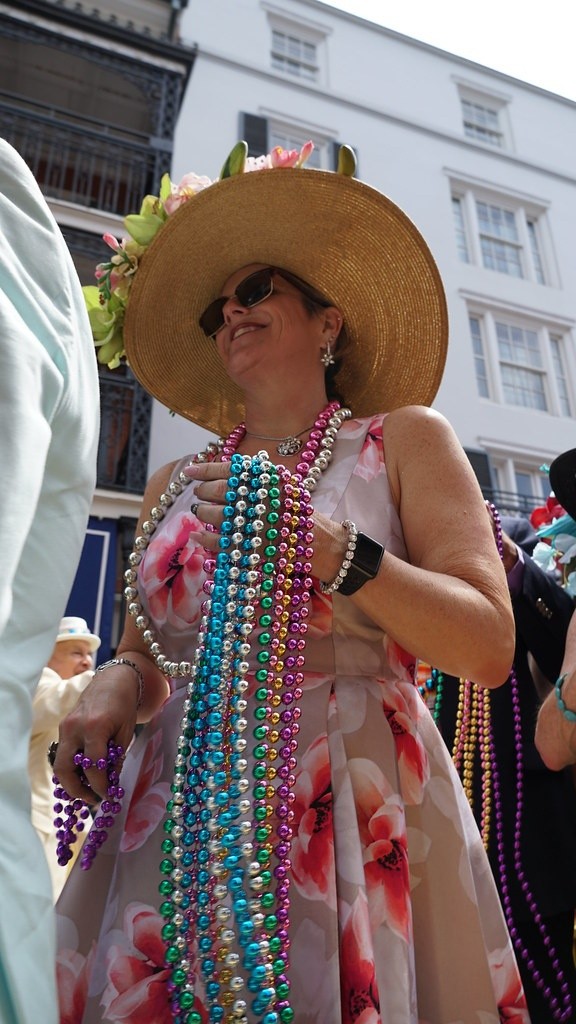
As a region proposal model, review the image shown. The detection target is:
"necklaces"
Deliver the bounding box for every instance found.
[54,403,354,1024]
[416,499,576,1024]
[246,426,312,456]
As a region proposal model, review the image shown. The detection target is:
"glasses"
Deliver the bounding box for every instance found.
[199,266,332,341]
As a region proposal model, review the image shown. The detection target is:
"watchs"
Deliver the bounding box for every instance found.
[336,531,384,596]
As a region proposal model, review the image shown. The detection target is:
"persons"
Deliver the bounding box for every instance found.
[0,136,98,1024]
[55,163,526,1024]
[533,449,575,770]
[30,616,101,898]
[437,499,575,1024]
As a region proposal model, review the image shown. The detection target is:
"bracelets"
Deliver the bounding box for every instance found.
[555,673,576,722]
[94,660,145,711]
[320,520,357,594]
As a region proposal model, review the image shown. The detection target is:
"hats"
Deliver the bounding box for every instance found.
[549,448,576,522]
[81,140,449,438]
[56,617,101,652]
